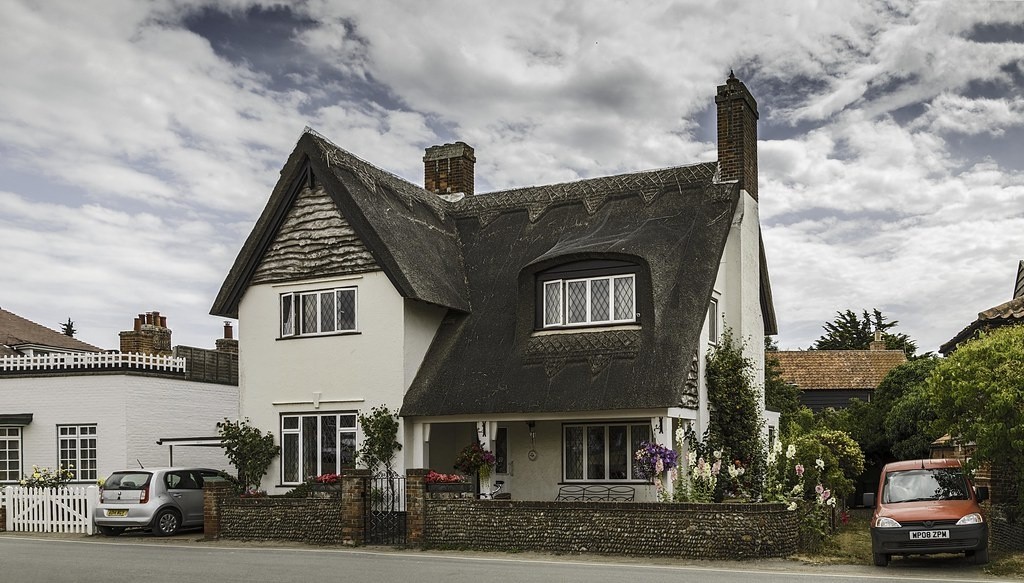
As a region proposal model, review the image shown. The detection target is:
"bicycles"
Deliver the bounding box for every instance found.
[476,483,511,499]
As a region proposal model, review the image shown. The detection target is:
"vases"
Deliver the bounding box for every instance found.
[427,482,471,493]
[312,482,341,491]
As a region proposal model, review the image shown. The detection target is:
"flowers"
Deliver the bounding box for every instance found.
[317,474,338,485]
[453,441,495,489]
[426,471,471,483]
[634,440,679,480]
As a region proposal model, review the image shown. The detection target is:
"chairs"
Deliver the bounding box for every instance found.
[555,486,636,502]
[175,476,197,489]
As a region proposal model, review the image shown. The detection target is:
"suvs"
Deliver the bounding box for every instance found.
[92,466,242,537]
[863,457,990,568]
